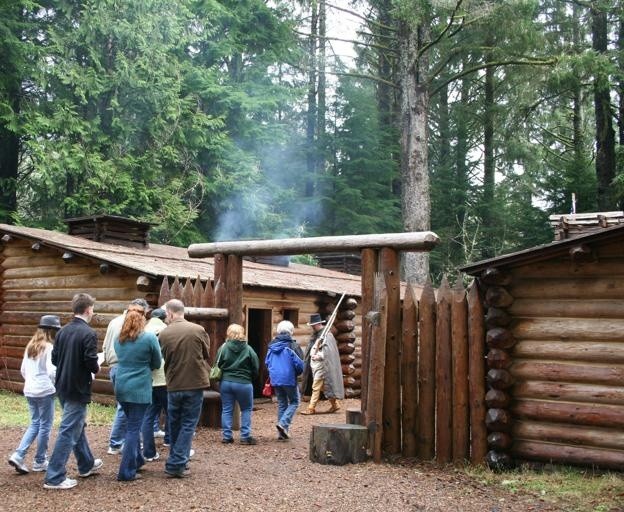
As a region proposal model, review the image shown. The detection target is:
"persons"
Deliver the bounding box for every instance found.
[300,313,340,415]
[213,323,259,445]
[265,320,304,440]
[7,315,62,474]
[102,298,211,482]
[42,293,103,489]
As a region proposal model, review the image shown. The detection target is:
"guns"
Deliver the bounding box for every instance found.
[313,293,346,355]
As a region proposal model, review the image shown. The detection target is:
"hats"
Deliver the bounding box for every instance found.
[39,314,61,329]
[307,313,326,326]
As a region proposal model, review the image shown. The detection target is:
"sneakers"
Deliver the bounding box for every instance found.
[143,451,160,462]
[221,437,234,444]
[275,424,290,441]
[31,459,78,491]
[300,408,316,415]
[78,459,103,478]
[239,436,258,445]
[7,452,29,474]
[163,469,192,479]
[329,403,340,412]
[153,429,166,438]
[106,446,123,455]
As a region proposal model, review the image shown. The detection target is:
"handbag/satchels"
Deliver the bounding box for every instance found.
[261,376,274,397]
[209,342,226,380]
